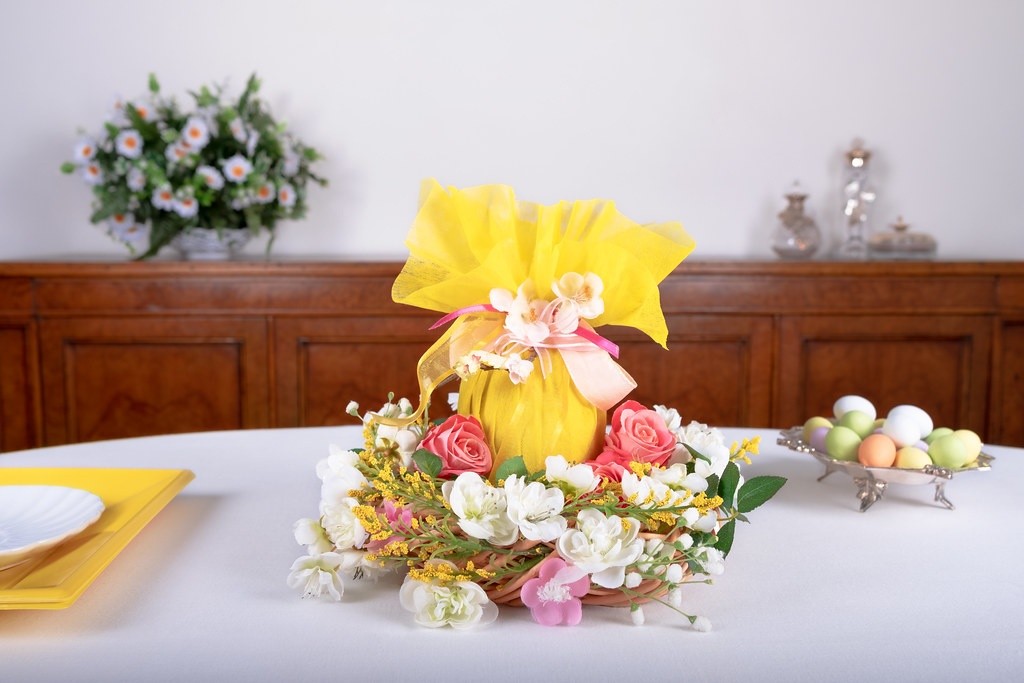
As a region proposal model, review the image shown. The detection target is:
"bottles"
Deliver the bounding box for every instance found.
[831,135,884,262]
[766,191,823,262]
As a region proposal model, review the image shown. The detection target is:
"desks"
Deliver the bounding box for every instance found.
[0,428,1024,683]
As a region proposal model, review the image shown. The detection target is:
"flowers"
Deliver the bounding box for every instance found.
[286,271,789,631]
[60,71,330,261]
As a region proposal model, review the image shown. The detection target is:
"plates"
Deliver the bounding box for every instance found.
[0,485,105,571]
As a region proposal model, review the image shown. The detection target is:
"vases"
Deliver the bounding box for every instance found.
[165,226,255,261]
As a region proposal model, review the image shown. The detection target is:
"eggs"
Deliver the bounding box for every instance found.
[802,395,982,468]
